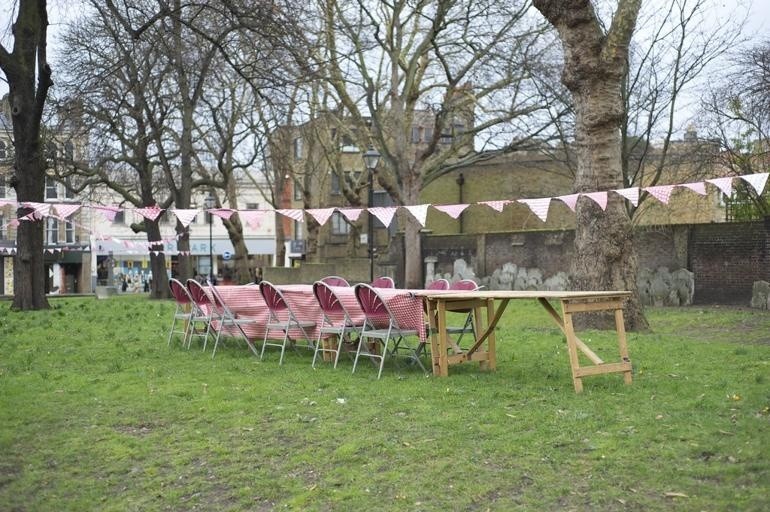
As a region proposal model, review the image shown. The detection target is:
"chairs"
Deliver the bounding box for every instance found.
[206,279,261,360]
[317,274,479,291]
[169,277,219,351]
[186,278,242,353]
[351,282,430,378]
[311,279,378,370]
[259,280,323,365]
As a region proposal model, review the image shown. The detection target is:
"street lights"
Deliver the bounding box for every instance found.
[362,143,382,283]
[205,191,217,281]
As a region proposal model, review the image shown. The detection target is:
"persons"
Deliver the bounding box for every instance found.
[119,269,152,292]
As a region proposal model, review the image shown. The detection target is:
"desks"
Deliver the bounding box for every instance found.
[432,290,634,393]
[201,285,476,363]
